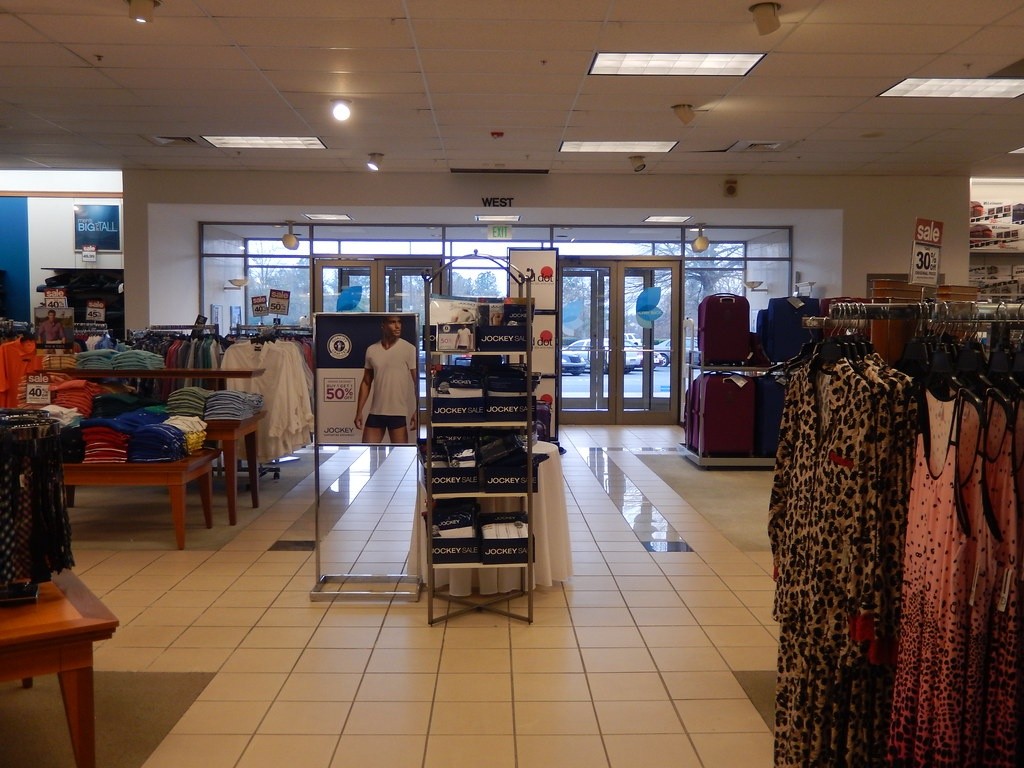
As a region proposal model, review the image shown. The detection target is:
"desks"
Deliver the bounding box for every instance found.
[0,569,120,768]
[60,447,222,550]
[198,411,267,526]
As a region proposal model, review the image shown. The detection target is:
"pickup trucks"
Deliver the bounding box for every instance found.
[564,339,641,374]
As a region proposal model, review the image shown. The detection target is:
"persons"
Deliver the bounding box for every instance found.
[454,325,471,350]
[354,317,419,443]
[39,310,66,355]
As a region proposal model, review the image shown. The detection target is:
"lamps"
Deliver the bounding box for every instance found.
[224,279,248,290]
[124,0,162,24]
[330,98,352,121]
[283,221,299,250]
[367,153,384,173]
[749,2,781,36]
[692,223,709,253]
[674,106,695,123]
[631,156,646,172]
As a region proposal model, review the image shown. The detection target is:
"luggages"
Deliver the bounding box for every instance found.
[682,292,872,457]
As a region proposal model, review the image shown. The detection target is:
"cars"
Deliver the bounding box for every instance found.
[626,333,642,345]
[623,341,664,370]
[654,337,701,367]
[562,350,586,375]
[419,336,473,372]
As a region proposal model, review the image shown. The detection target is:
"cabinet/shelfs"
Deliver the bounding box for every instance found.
[421,266,534,629]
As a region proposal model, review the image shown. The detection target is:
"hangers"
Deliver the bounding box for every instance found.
[805,300,1024,412]
[701,365,785,384]
[73,323,313,356]
[0,410,59,470]
